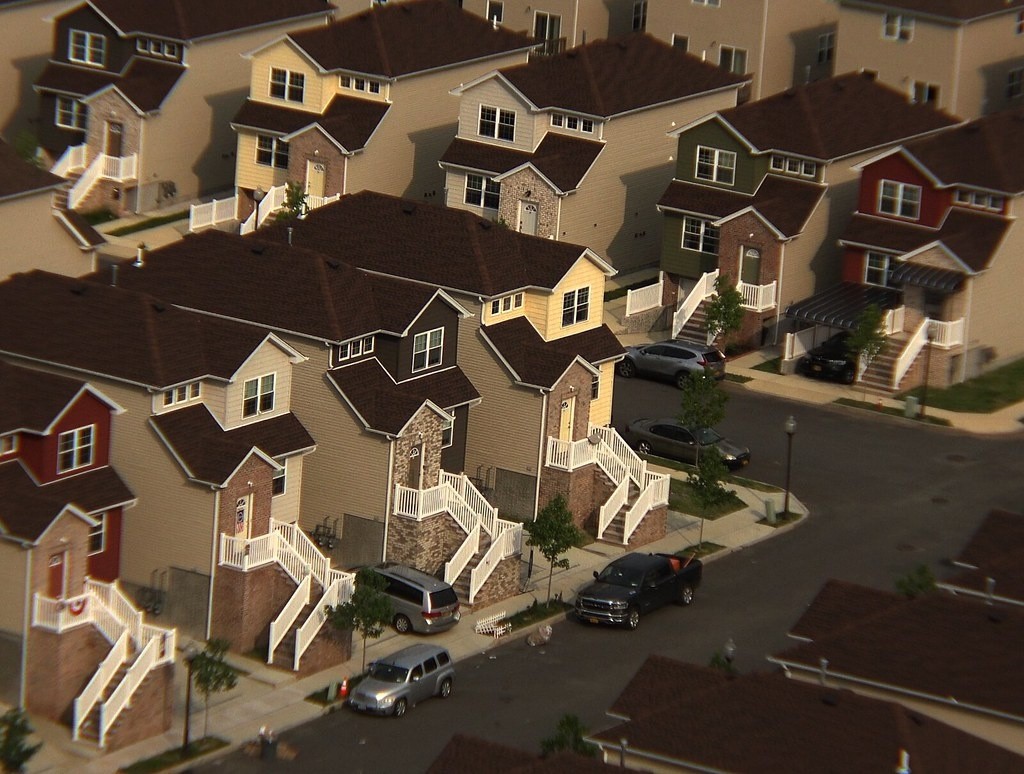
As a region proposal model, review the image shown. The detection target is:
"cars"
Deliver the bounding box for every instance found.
[798,330,872,385]
[625,417,751,472]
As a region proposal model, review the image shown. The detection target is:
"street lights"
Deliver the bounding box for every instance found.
[181,639,199,754]
[920,322,938,420]
[785,415,796,520]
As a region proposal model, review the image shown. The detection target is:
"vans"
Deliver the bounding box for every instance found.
[346,562,462,635]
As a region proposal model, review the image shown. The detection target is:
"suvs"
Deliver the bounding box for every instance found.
[349,642,456,718]
[615,338,727,391]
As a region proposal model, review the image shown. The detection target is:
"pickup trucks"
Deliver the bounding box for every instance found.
[574,553,704,628]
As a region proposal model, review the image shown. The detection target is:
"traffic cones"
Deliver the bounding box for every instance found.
[339,676,347,696]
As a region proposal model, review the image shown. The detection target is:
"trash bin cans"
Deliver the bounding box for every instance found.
[259,735,278,760]
[905,396,919,419]
[765,498,776,524]
